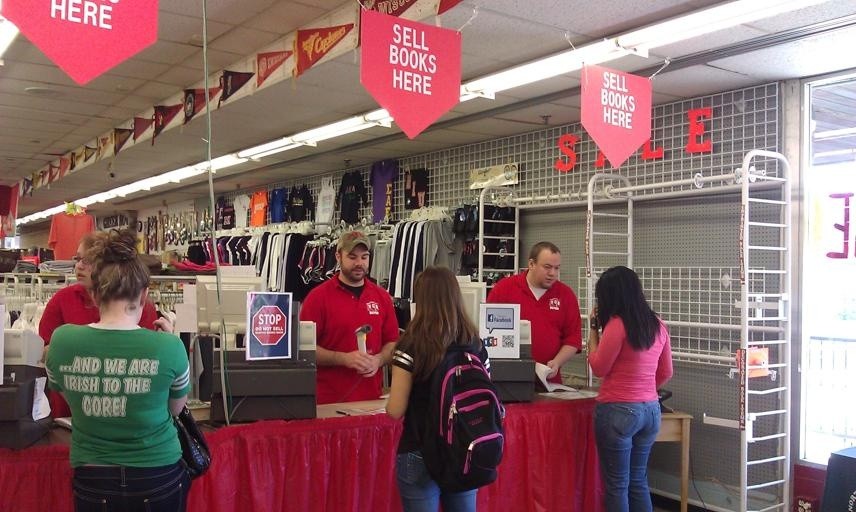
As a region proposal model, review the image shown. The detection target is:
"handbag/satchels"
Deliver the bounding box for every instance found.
[171,403,212,476]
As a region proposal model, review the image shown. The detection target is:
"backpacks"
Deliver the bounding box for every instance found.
[397,334,506,493]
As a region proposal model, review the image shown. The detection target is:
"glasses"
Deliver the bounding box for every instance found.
[72,256,89,264]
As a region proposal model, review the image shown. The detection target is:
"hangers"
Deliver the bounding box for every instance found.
[218,205,451,241]
[0,290,184,318]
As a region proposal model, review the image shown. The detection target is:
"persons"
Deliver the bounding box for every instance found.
[46,225,193,512]
[588,266,673,512]
[386,266,491,512]
[488,242,582,391]
[38,232,158,422]
[299,232,400,405]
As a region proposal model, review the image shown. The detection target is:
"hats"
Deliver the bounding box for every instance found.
[337,232,371,255]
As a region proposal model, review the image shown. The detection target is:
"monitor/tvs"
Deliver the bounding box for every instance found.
[194,275,267,350]
[458,281,487,332]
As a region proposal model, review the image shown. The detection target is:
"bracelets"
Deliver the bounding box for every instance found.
[590,317,599,328]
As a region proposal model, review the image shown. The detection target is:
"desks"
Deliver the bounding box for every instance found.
[649,409,694,512]
